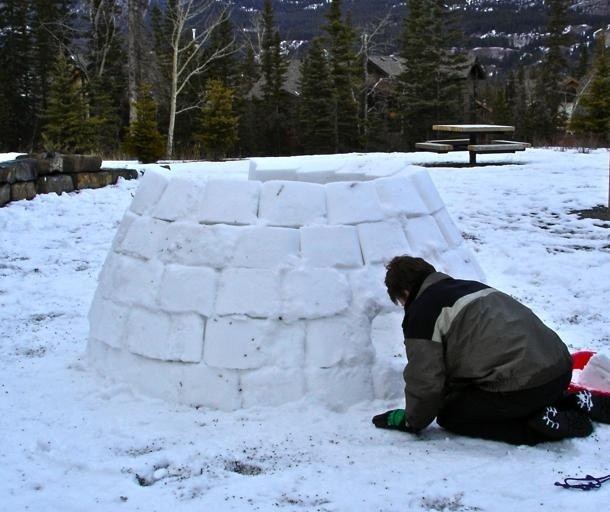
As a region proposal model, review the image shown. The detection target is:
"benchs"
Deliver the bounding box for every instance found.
[415,122,532,163]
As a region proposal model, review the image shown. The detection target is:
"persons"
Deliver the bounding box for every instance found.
[371,252,596,447]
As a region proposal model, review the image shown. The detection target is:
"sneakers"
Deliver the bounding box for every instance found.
[541,406,593,439]
[569,390,595,412]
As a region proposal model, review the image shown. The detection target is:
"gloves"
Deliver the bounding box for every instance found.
[371,408,420,434]
